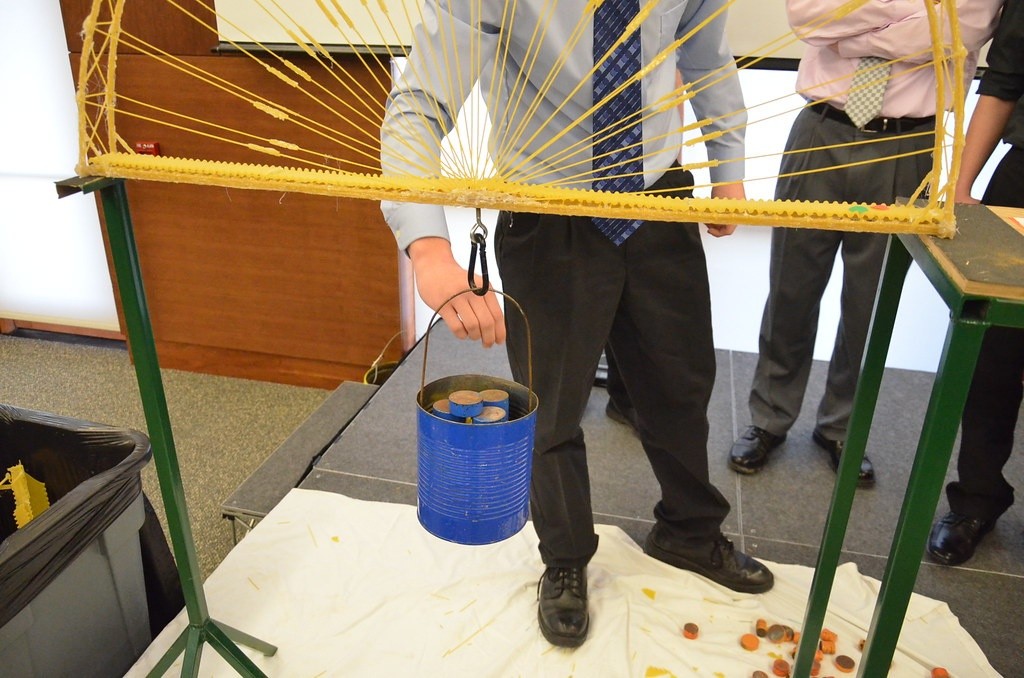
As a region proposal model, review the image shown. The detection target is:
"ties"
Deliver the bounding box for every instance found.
[593,0,644,246]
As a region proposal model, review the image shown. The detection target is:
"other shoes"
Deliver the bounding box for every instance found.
[605,403,630,425]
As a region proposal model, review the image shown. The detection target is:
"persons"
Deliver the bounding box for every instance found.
[376,0,774,652]
[926,0,1024,567]
[727,0,1004,487]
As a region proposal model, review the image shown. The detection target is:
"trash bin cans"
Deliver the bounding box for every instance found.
[0,405,155,678]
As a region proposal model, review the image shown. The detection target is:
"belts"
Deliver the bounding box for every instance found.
[806,99,935,134]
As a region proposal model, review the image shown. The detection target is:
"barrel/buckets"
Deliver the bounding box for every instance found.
[415,288,539,546]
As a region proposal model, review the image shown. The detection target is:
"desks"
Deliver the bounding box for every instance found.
[788,196,1024,678]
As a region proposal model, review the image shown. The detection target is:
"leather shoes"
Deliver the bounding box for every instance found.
[812,431,876,488]
[643,533,774,594]
[728,425,787,476]
[537,567,589,647]
[925,510,997,567]
